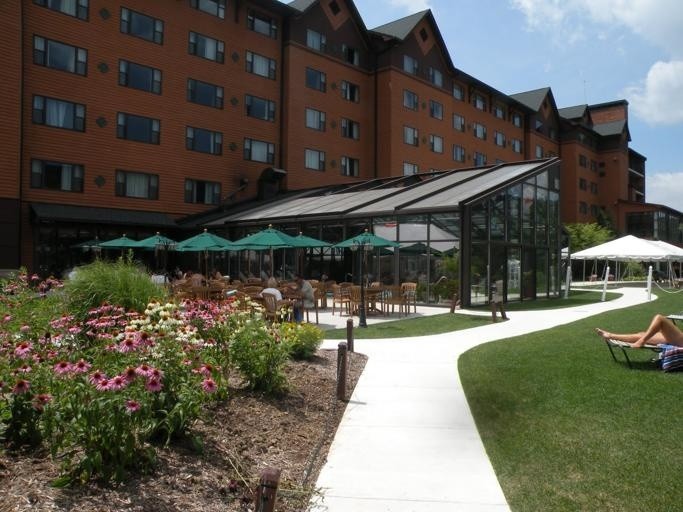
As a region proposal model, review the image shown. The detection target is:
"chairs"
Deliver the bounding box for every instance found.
[193,278,421,324]
[604,338,683,371]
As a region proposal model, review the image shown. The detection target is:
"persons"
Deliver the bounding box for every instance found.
[596,314,683,348]
[259,277,293,322]
[294,276,314,324]
